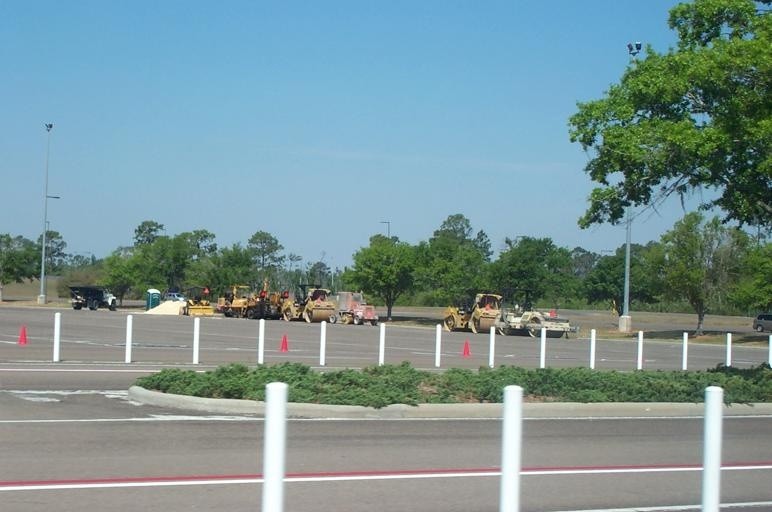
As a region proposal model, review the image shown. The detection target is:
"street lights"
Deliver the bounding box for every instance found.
[37,121,59,305]
[380,220,391,238]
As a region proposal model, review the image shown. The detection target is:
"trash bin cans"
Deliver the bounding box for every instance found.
[146,289,161,311]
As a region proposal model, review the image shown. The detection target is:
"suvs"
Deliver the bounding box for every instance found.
[751,311,771,332]
[165,294,188,302]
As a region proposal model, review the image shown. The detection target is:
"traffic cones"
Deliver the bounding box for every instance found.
[280,335,292,352]
[17,326,29,346]
[461,339,472,358]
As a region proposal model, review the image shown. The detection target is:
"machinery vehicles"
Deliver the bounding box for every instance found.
[441,287,578,343]
[69,283,117,312]
[183,276,383,327]
